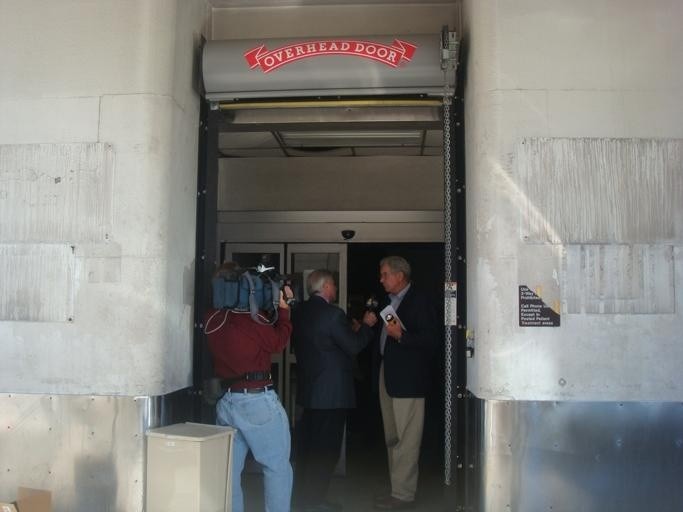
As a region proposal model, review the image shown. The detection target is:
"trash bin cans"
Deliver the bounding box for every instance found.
[145,421,238,512]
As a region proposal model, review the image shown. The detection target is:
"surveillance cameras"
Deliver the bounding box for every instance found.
[341,230,355,239]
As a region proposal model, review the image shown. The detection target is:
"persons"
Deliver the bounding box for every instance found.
[201,260,295,511]
[350,253,439,512]
[282,269,377,511]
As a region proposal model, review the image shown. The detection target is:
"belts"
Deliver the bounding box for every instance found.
[226,385,276,394]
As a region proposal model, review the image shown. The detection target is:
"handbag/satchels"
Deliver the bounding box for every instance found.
[201,376,226,405]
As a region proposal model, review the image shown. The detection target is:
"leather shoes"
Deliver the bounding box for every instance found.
[304,499,342,512]
[375,491,416,511]
